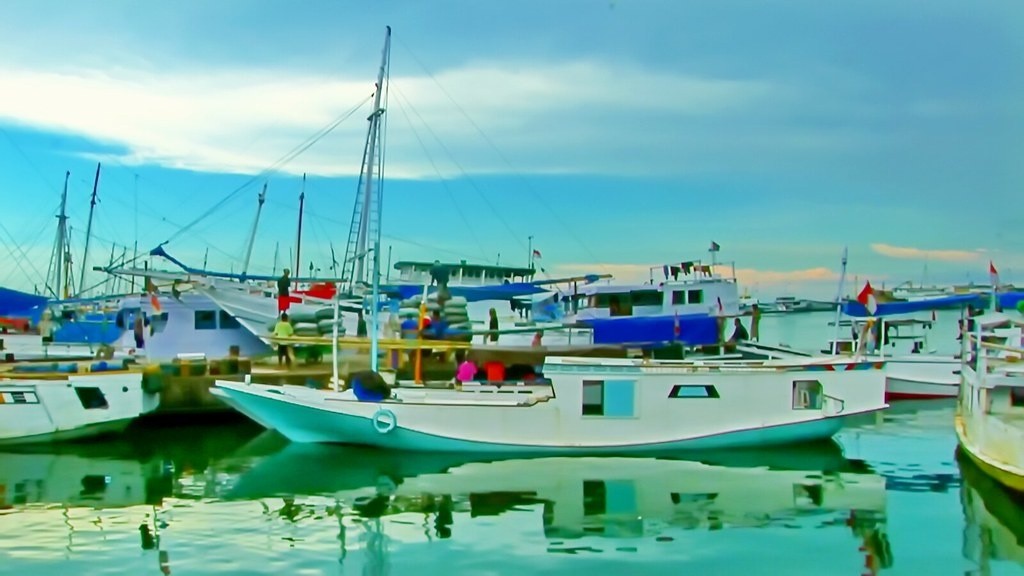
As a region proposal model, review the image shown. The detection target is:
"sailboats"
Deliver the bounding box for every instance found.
[89,22,890,457]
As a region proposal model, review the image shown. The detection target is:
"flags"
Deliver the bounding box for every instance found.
[533,251,541,258]
[857,282,877,314]
[713,243,719,250]
[989,264,998,283]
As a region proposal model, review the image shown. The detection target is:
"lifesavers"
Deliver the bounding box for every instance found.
[864,321,874,337]
[96,348,110,360]
[373,409,396,434]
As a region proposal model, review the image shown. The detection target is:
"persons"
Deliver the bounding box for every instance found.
[750,305,761,344]
[427,310,448,340]
[401,313,418,328]
[728,318,749,343]
[278,269,291,297]
[274,313,294,365]
[532,331,543,346]
[490,308,498,342]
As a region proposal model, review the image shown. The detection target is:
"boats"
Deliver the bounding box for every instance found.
[1,161,1024,445]
[951,285,1023,500]
